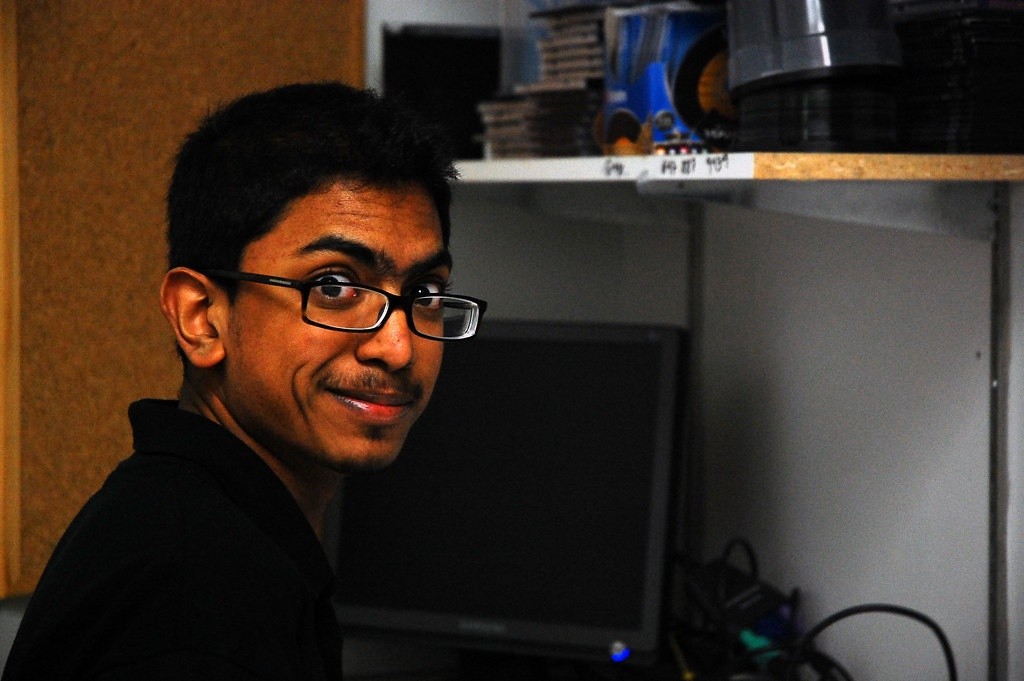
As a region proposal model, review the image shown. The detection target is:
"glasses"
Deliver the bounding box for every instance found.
[200,268,487,342]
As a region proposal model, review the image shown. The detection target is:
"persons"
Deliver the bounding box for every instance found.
[1,80,454,680]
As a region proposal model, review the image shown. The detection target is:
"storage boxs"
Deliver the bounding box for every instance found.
[604,2,737,154]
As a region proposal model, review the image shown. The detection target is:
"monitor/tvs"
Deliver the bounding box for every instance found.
[324,319,690,666]
